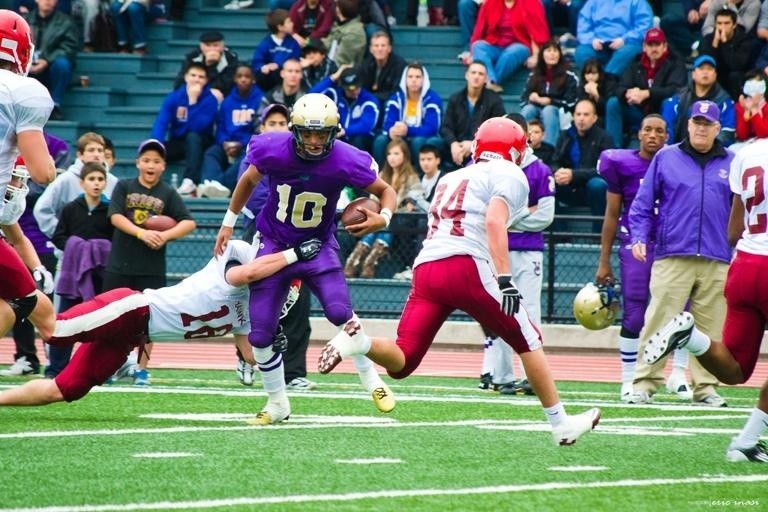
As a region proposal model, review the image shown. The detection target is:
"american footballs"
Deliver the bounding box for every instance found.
[342,197,382,232]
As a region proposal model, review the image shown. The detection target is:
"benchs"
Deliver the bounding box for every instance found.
[0,1,768,324]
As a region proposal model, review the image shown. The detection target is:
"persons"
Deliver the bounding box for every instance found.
[0,0,717,278]
[621,100,738,406]
[234,101,321,391]
[104,139,197,388]
[642,137,767,462]
[594,112,691,401]
[478,113,556,395]
[0,230,324,408]
[41,161,112,379]
[318,116,601,447]
[212,92,397,426]
[31,132,121,361]
[1,6,57,340]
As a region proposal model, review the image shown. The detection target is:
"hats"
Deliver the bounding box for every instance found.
[341,69,361,86]
[199,32,223,42]
[645,28,665,44]
[139,138,167,157]
[693,54,717,68]
[691,99,720,122]
[302,36,328,54]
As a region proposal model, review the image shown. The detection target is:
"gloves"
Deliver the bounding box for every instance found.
[271,325,288,353]
[293,236,322,261]
[33,265,54,295]
[497,274,523,317]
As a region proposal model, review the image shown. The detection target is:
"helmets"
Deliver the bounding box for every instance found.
[0,8,35,77]
[573,281,622,330]
[279,278,302,319]
[287,92,341,161]
[470,116,528,166]
[0,155,31,225]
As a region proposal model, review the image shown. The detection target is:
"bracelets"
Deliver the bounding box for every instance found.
[281,247,299,266]
[135,228,145,240]
[381,213,391,228]
[221,208,238,229]
[380,208,393,219]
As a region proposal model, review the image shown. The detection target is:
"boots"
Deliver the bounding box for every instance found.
[342,240,389,278]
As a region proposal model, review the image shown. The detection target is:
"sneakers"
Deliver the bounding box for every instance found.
[134,369,150,385]
[236,346,314,426]
[553,407,600,445]
[175,177,230,197]
[317,319,363,375]
[620,374,727,407]
[727,436,768,464]
[1,355,35,374]
[360,368,396,412]
[480,373,534,396]
[644,311,694,365]
